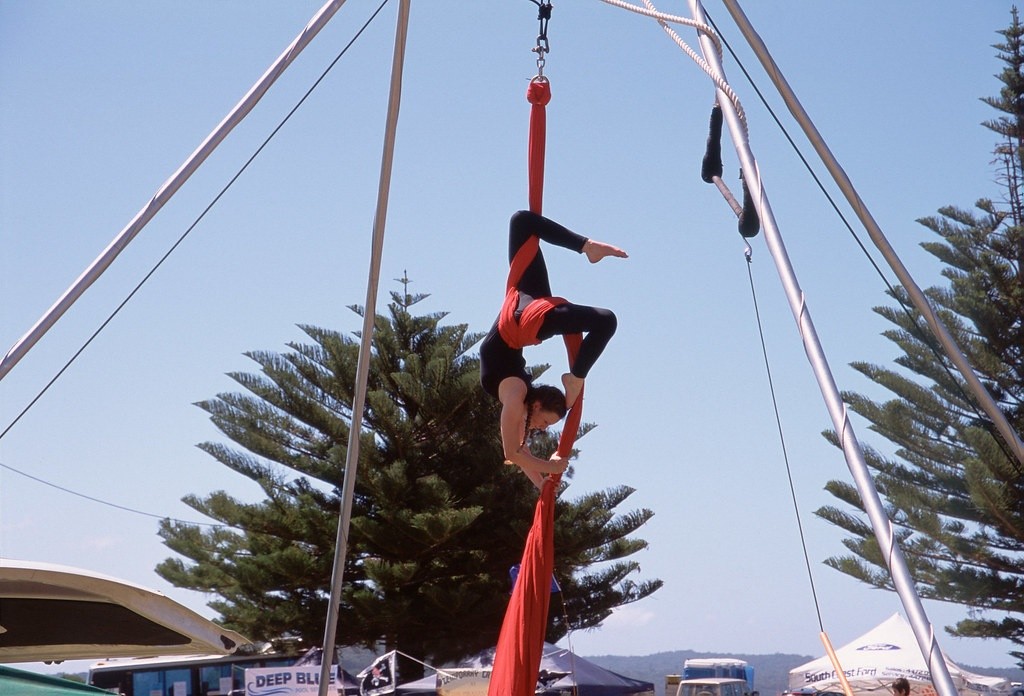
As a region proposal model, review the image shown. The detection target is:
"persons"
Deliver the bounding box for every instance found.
[891,678,910,696]
[479,210,627,502]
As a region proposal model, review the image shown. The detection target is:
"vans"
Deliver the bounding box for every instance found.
[676,679,759,696]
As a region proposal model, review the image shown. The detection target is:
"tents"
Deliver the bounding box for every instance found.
[395,641,655,696]
[788,610,1016,696]
